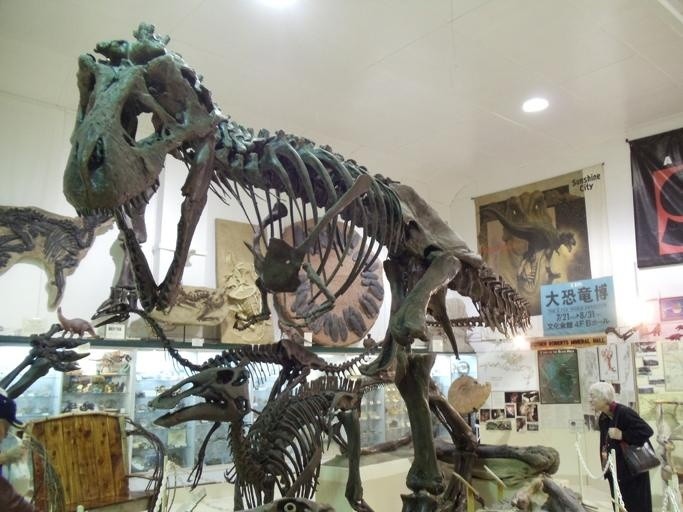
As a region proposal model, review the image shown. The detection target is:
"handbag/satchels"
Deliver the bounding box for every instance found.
[620,440,660,475]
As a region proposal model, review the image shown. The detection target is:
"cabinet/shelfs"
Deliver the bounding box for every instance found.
[53,338,480,512]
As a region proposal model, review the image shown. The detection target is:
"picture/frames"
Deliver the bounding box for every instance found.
[504,403,517,419]
[515,415,527,433]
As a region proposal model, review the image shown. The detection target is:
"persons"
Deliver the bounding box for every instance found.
[0,393,41,511]
[588,382,655,512]
